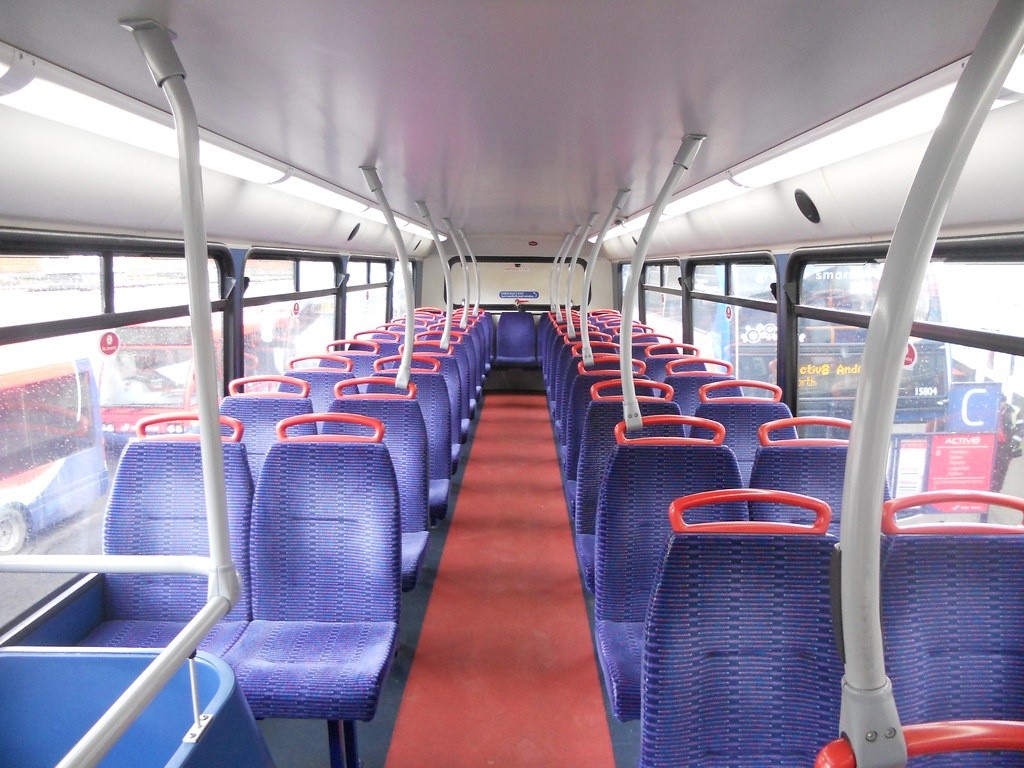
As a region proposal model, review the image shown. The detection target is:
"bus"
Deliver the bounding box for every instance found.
[1,355,110,556]
[622,260,951,437]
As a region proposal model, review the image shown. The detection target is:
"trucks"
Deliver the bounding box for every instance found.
[98,298,299,460]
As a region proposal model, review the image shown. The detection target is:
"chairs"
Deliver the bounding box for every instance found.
[72,305,1024,768]
